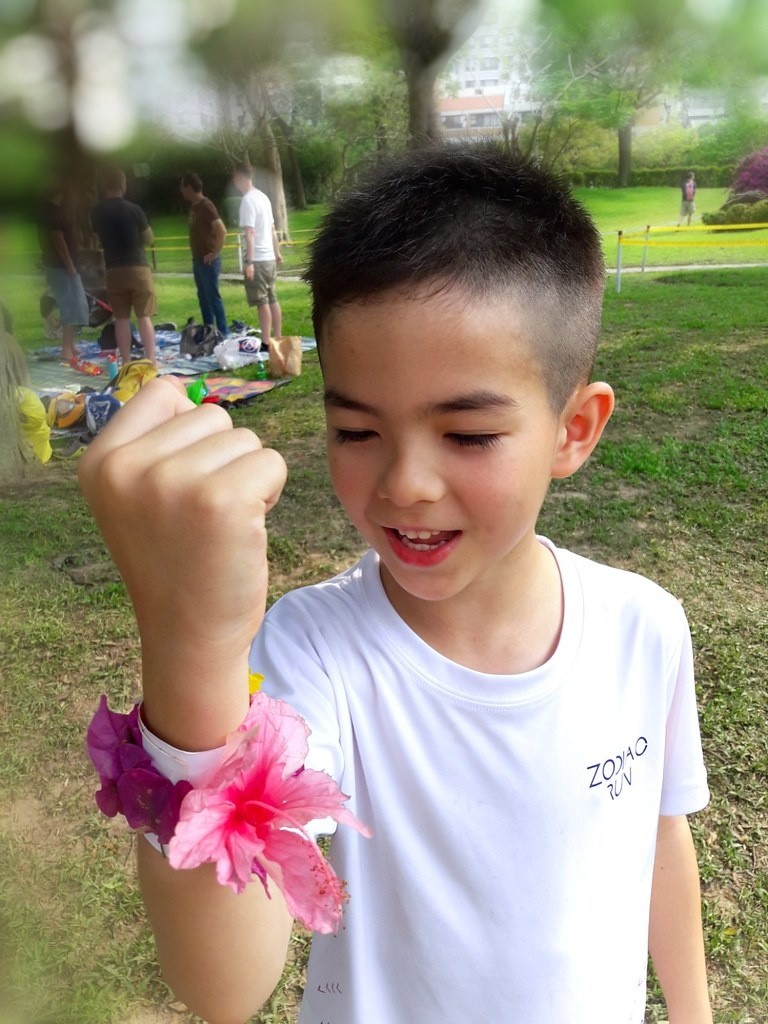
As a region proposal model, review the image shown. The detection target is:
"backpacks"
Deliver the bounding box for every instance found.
[99,359,159,409]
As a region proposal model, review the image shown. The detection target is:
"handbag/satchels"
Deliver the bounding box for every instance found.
[97,318,144,351]
[178,324,224,358]
[267,335,303,378]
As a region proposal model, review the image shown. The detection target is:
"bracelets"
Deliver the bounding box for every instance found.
[86,667,372,939]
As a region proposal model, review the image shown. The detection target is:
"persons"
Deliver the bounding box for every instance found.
[80,141,713,1024]
[235,164,284,350]
[90,165,156,368]
[678,171,696,227]
[38,185,89,361]
[180,171,230,338]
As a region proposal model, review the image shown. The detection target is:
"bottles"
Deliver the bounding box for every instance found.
[255,360,267,380]
[106,354,118,380]
